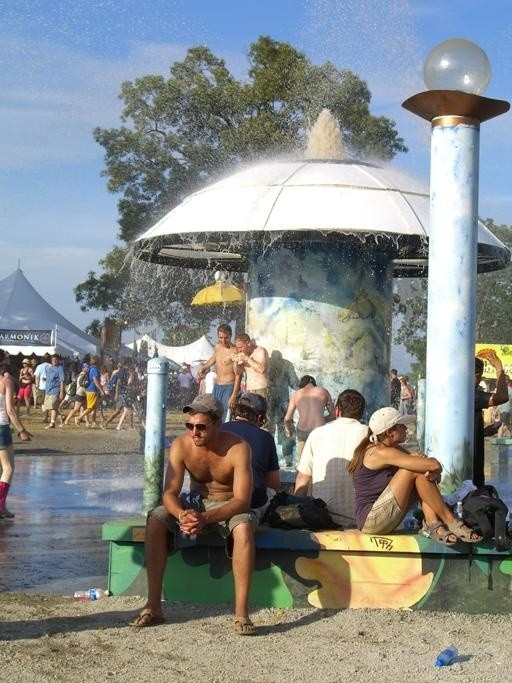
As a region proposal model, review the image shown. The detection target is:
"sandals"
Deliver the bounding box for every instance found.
[445,520,483,544]
[419,518,459,546]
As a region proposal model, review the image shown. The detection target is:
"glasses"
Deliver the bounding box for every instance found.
[185,420,215,431]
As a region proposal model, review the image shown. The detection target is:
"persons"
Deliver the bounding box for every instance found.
[0,349,31,518]
[390,368,413,416]
[347,405,482,546]
[166,361,217,411]
[200,323,243,424]
[292,389,369,529]
[284,374,337,497]
[219,393,281,522]
[232,331,269,398]
[130,392,258,635]
[5,354,147,430]
[473,351,508,486]
[496,374,512,436]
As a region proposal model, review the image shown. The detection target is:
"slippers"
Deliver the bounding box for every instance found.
[234,616,256,635]
[128,608,164,627]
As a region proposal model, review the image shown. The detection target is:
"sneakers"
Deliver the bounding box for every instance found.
[1,509,14,518]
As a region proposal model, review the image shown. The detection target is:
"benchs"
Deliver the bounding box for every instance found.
[101,508,509,613]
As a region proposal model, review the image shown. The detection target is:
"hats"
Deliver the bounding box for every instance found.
[22,358,31,365]
[183,393,224,418]
[370,406,417,436]
[238,393,268,413]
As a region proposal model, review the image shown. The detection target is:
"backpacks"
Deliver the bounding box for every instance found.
[462,485,511,552]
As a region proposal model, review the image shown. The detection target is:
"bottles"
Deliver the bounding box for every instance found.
[454,501,464,522]
[73,588,111,600]
[397,518,418,532]
[436,646,460,667]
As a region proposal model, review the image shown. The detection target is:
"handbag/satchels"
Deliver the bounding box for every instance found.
[79,372,90,387]
[263,491,342,530]
[65,383,76,396]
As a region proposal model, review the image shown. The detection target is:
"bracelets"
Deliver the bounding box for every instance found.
[494,368,505,373]
[18,428,27,434]
[178,508,185,521]
